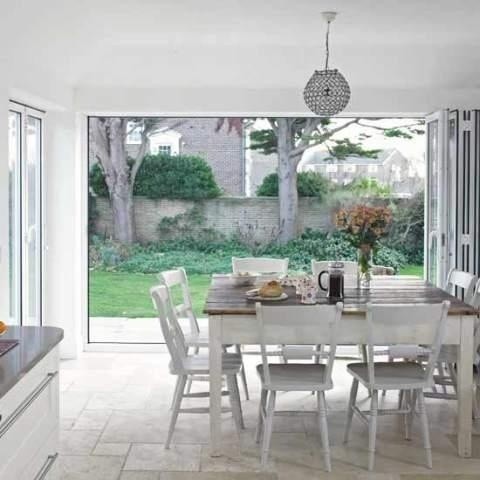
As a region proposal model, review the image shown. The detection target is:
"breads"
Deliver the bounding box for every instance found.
[258,281,283,298]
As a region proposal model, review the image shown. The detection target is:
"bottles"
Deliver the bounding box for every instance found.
[318,261,346,301]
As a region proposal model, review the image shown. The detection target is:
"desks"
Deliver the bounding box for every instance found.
[203,275,480,469]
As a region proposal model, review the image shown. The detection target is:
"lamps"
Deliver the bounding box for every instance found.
[302,10,352,117]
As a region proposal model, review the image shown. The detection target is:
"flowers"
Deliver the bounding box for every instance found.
[335,206,392,275]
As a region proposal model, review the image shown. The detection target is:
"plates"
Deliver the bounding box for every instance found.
[246,289,288,302]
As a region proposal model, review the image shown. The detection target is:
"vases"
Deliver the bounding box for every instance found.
[357,247,372,290]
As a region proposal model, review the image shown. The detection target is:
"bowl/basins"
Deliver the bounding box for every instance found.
[228,272,258,288]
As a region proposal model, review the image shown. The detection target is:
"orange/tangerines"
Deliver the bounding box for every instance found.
[0,321,6,333]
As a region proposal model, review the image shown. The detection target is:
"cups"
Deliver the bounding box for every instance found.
[294,275,320,308]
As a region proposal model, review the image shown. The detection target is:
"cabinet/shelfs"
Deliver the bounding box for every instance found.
[0,322,64,479]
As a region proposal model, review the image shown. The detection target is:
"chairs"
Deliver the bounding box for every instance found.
[310,258,364,385]
[350,300,447,473]
[151,285,244,448]
[252,303,345,470]
[231,255,291,397]
[382,267,477,413]
[158,266,249,404]
